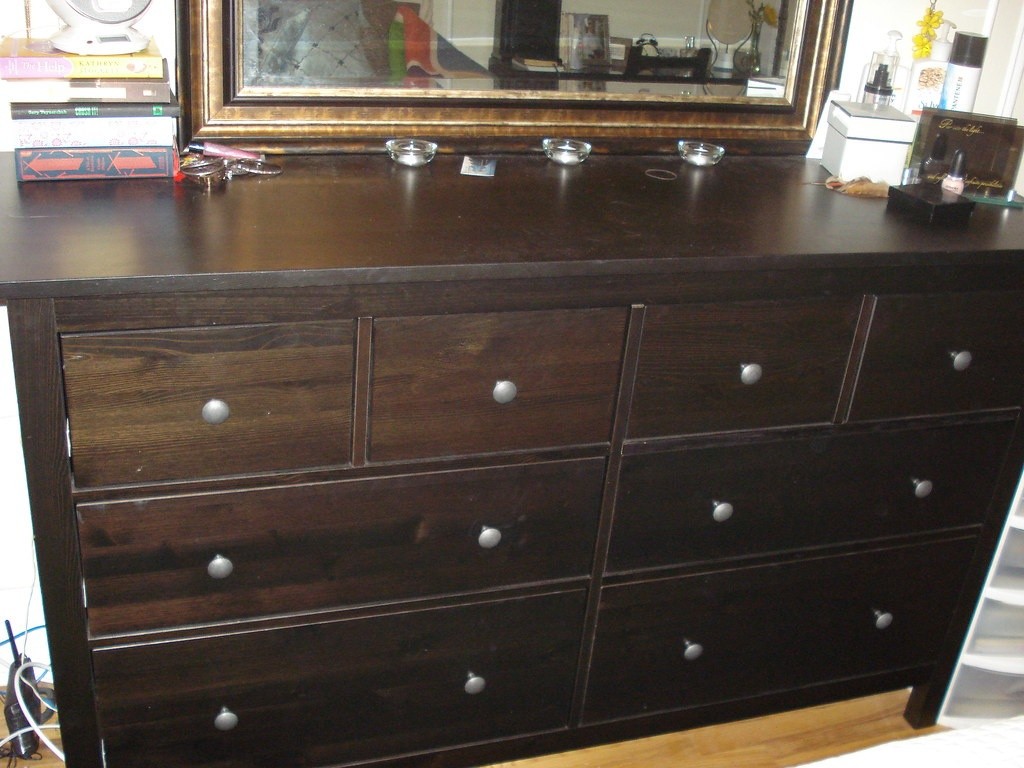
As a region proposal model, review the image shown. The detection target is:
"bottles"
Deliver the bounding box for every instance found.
[683,36,695,78]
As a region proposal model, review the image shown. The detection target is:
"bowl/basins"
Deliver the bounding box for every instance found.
[387,138,438,166]
[678,141,725,167]
[543,138,592,165]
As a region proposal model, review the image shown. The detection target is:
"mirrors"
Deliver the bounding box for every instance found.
[174,0,852,153]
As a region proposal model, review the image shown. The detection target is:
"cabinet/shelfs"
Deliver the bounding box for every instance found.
[0,150,1024,768]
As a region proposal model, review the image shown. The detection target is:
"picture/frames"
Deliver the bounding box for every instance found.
[568,14,612,67]
